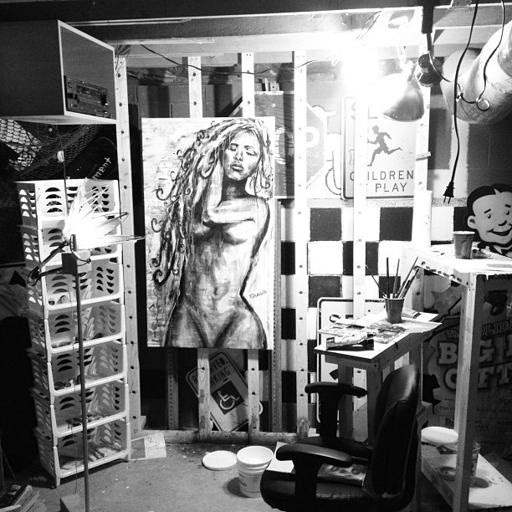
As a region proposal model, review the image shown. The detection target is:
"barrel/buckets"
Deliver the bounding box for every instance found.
[421,426,458,474]
[237,446,273,498]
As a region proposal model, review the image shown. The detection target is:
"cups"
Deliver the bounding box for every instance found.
[383,294,406,323]
[453,230,475,259]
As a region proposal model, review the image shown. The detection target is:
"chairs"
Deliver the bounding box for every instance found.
[256,362,420,511]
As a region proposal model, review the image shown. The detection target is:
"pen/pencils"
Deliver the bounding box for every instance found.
[364,256,420,299]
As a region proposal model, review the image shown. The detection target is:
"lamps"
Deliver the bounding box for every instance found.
[378,1,448,123]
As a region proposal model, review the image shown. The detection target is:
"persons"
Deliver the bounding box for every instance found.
[149,117,274,350]
[465,181,512,260]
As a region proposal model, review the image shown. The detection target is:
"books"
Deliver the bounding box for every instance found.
[318,317,402,344]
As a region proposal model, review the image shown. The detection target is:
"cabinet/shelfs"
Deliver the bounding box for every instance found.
[0,19,120,126]
[14,176,139,487]
